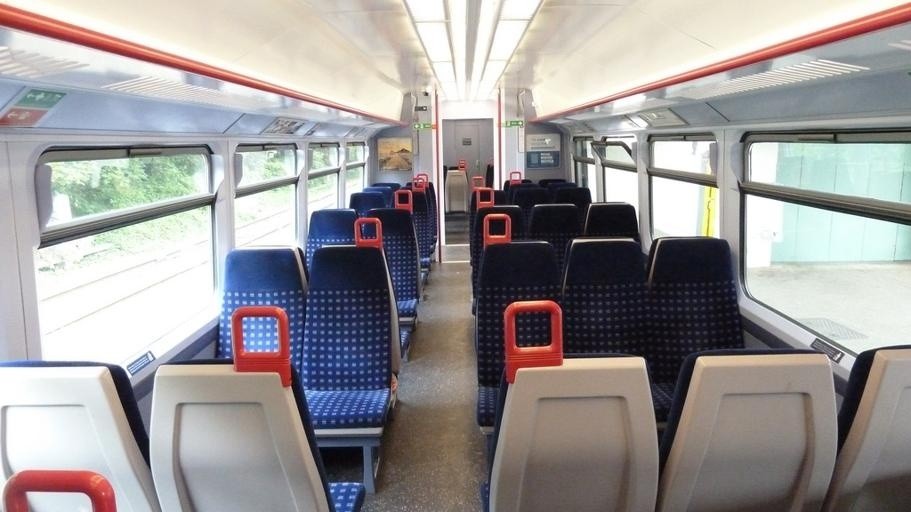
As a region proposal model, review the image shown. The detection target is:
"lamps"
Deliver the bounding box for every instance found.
[400,0,543,98]
